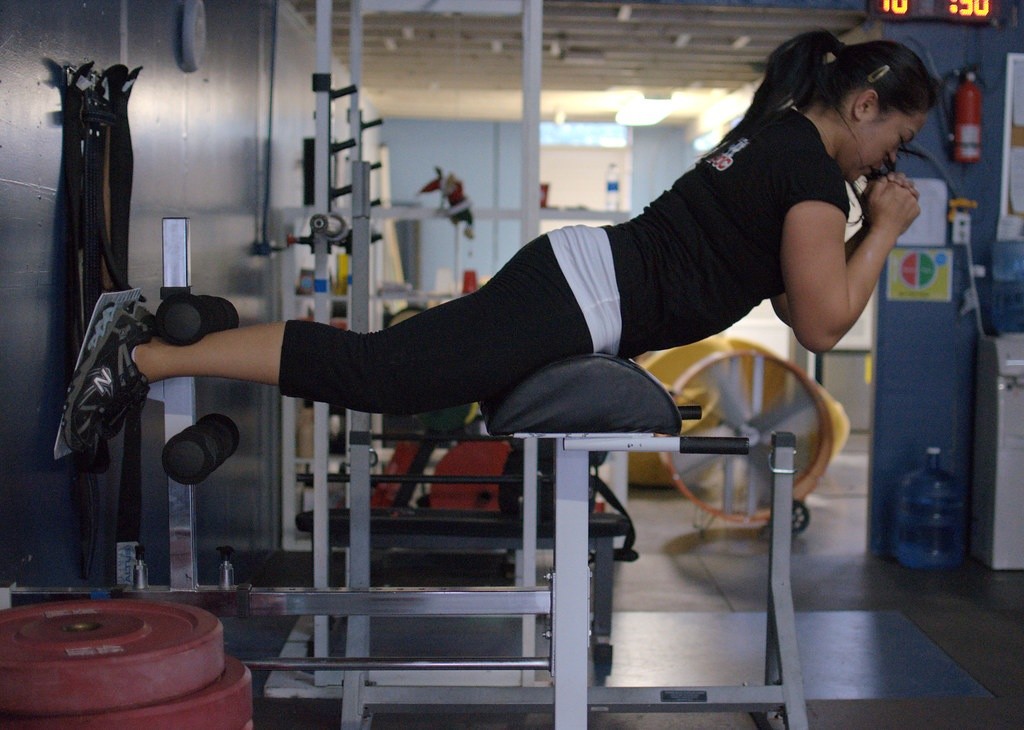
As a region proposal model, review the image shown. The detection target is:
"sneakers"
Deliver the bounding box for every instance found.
[62,303,151,454]
[95,301,154,438]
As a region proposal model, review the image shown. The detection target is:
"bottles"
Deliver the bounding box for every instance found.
[895,448,967,569]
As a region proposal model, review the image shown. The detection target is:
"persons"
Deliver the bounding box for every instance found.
[60,26,938,459]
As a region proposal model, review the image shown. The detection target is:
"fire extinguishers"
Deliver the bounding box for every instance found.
[938,65,985,166]
